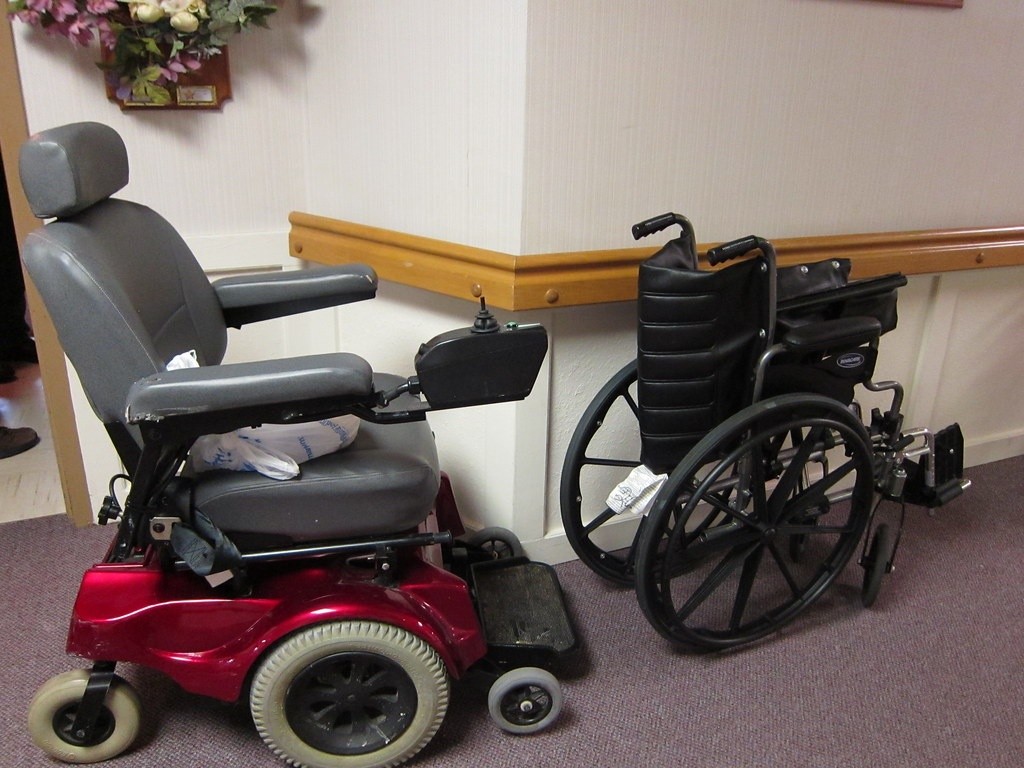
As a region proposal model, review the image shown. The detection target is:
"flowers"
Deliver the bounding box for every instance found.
[3,0,277,105]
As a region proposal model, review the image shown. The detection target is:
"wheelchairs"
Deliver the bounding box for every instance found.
[558,213,972,652]
[16,116,589,767]
